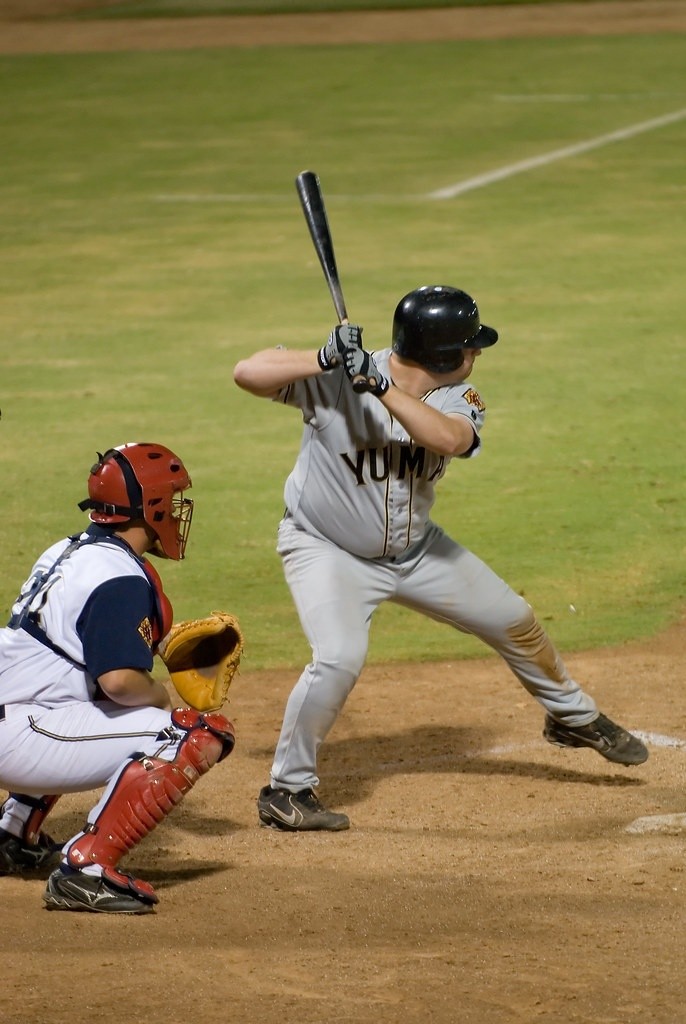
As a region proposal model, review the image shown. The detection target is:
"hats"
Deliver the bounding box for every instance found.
[76,442,202,562]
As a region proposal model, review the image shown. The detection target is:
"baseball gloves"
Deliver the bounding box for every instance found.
[157,610,245,711]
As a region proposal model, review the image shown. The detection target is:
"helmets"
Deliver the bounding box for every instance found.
[391,283,499,376]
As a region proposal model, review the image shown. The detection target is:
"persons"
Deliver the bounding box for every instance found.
[234,283,648,831]
[0,440,234,915]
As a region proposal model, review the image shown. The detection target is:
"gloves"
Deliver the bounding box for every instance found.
[345,349,390,396]
[317,321,365,372]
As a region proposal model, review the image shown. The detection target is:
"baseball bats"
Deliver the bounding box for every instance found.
[295,169,372,394]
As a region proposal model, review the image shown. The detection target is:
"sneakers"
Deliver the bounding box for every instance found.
[0,826,75,876]
[542,709,649,767]
[41,859,157,917]
[257,783,351,832]
